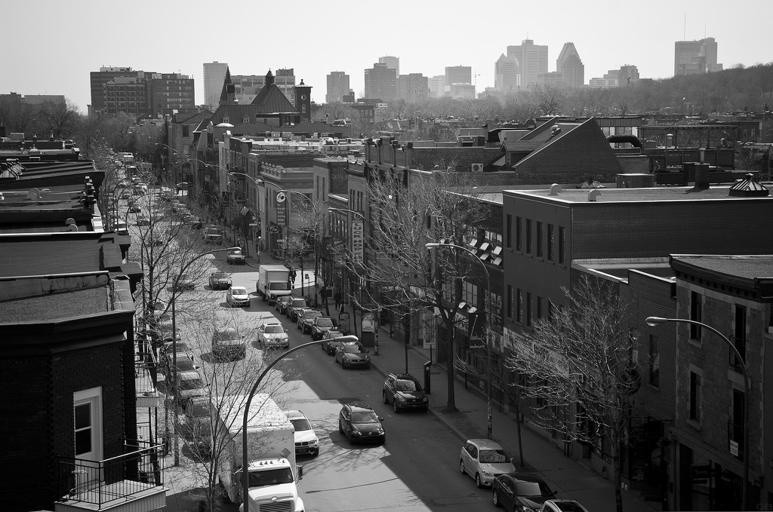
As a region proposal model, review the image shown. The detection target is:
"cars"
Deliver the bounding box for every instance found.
[96,142,135,167]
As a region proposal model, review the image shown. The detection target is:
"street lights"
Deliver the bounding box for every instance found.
[425,243,494,442]
[646,316,749,511]
[101,162,190,355]
